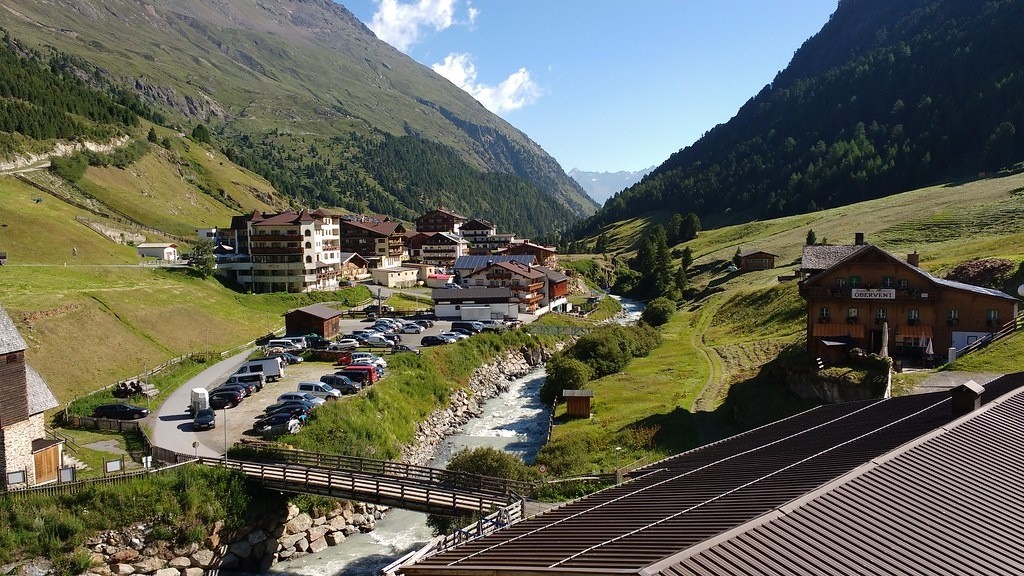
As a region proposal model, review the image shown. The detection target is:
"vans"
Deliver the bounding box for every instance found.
[223,371,267,392]
[231,355,286,383]
[268,339,302,352]
[281,336,308,351]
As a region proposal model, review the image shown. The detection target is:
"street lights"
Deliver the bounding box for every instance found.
[143,360,150,409]
[205,328,208,360]
[223,405,232,471]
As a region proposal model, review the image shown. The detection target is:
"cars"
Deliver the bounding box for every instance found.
[343,304,510,358]
[208,391,242,410]
[252,351,387,439]
[266,354,291,365]
[283,352,304,364]
[219,381,257,397]
[306,336,333,349]
[338,281,352,287]
[208,385,247,399]
[95,403,149,421]
[193,407,218,431]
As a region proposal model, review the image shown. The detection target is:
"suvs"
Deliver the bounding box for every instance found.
[328,338,360,350]
